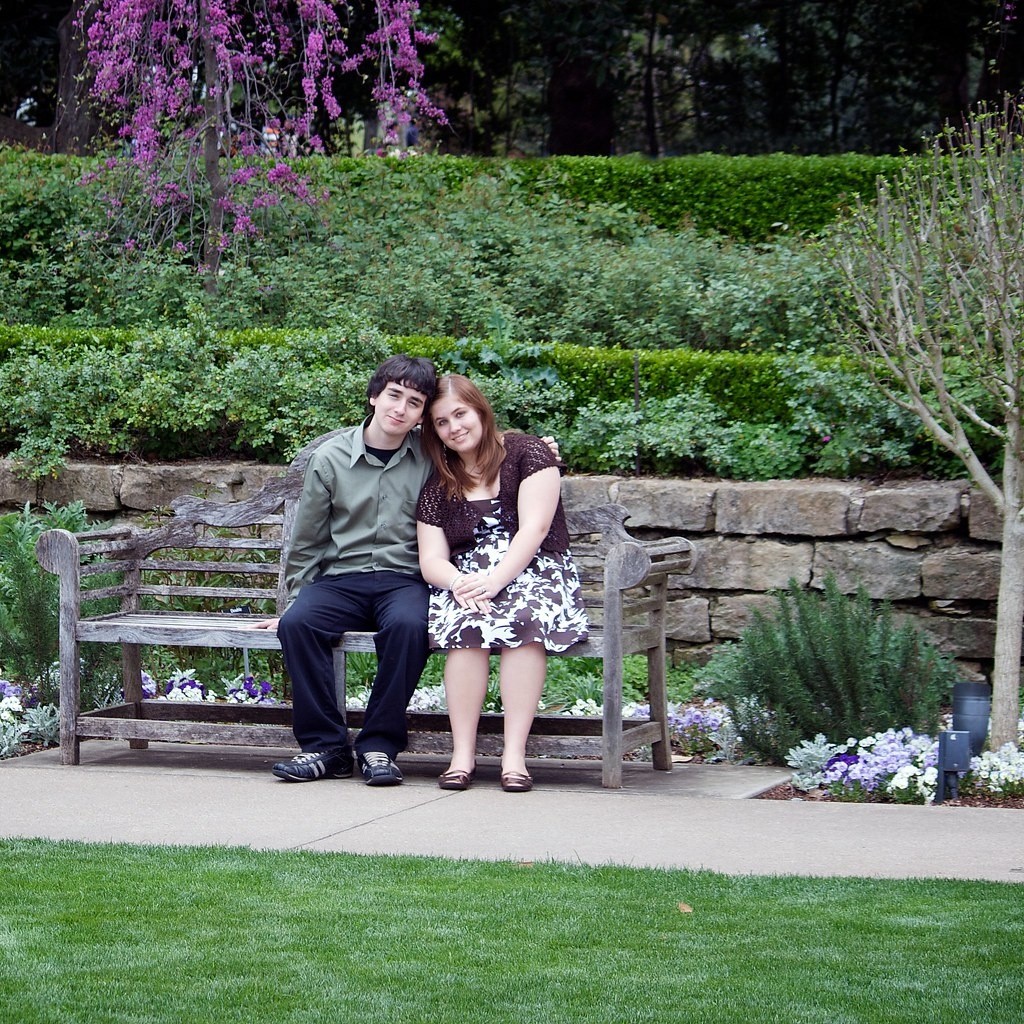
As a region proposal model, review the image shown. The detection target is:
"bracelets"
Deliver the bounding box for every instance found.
[450,574,465,590]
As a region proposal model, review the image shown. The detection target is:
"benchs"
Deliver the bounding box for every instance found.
[37,425,697,788]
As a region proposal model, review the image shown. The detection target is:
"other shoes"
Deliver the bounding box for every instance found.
[438,759,477,790]
[500,755,533,791]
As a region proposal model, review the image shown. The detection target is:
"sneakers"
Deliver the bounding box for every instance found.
[358,751,404,785]
[272,744,355,781]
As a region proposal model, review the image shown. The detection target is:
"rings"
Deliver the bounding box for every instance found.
[481,586,486,594]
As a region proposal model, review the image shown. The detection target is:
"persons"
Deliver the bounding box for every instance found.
[247,354,589,791]
